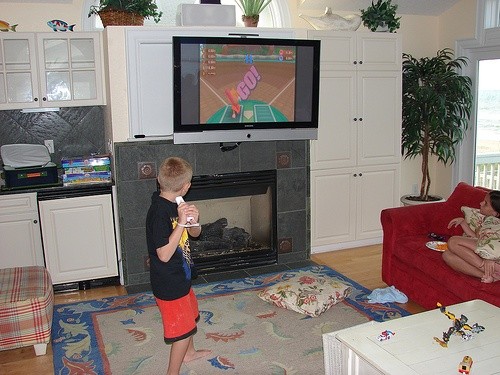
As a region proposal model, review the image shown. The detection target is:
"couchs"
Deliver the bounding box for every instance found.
[381,181,500,310]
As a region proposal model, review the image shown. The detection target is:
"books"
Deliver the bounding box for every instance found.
[62,156,112,185]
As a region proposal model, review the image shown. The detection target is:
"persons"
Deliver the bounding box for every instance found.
[441,191,500,283]
[146,156,212,375]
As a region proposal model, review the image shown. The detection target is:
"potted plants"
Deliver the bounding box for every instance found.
[358,0,401,33]
[88,0,163,28]
[400,47,474,206]
[234,0,272,27]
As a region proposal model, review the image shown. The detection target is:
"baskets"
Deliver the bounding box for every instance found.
[97,11,145,28]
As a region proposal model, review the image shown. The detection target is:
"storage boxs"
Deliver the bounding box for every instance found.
[0,144,57,186]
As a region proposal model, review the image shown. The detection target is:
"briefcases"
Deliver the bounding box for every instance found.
[0,143,58,188]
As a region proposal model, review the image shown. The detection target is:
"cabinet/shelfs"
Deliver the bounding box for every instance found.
[0,29,107,114]
[308,31,399,250]
[0,192,119,293]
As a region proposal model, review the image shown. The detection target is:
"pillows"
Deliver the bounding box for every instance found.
[256,272,351,318]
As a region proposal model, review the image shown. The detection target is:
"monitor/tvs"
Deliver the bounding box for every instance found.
[172,36,321,143]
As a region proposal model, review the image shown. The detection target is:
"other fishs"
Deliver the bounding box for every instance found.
[47,20,76,32]
[0,20,18,32]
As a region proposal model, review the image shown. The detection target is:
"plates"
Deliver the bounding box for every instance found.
[426,241,447,251]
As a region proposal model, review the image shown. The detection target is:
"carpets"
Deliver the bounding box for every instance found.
[51,265,413,375]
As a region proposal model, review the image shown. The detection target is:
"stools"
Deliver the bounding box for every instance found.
[0,266,54,357]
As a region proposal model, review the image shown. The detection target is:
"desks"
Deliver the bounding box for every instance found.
[335,298,500,375]
